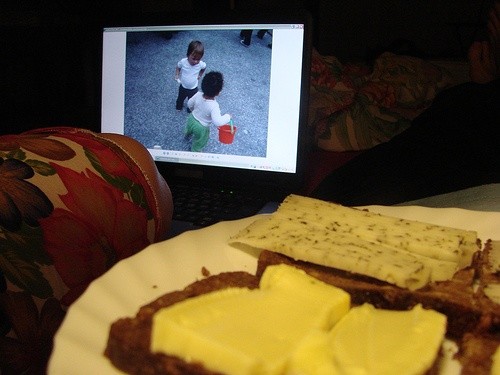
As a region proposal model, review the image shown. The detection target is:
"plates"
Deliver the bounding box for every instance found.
[46,205,500,375]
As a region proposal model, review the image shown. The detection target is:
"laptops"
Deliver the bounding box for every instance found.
[97,21,311,241]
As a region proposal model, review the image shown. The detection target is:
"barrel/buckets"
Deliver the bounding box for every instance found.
[217,118,237,145]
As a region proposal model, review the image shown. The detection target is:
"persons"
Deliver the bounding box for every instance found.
[182,70,233,153]
[239,30,252,47]
[174,39,207,112]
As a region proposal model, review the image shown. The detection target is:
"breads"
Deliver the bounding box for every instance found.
[99,251,499,375]
